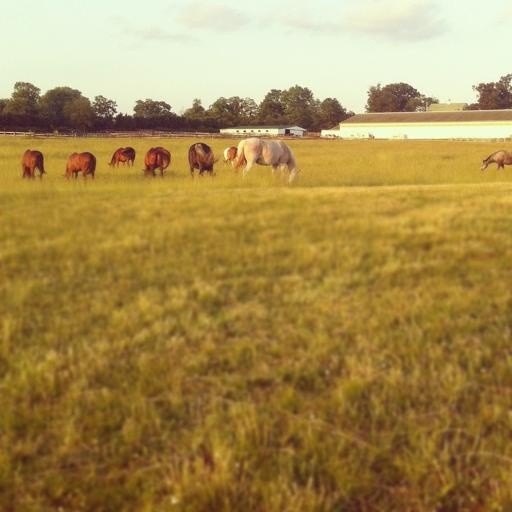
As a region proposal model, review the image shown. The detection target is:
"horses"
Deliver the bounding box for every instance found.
[21,149,45,179]
[65,152,96,180]
[481,149,512,172]
[108,147,135,167]
[188,142,214,179]
[224,145,237,163]
[144,146,171,177]
[233,137,302,184]
